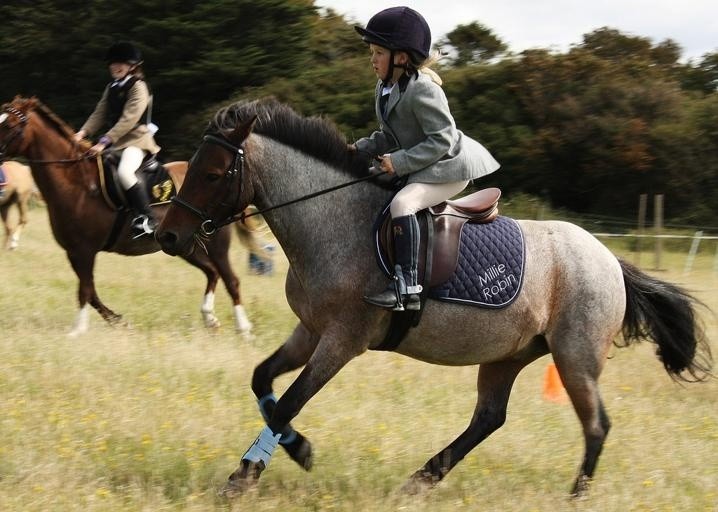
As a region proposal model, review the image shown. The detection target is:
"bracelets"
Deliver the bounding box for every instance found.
[99,137,110,145]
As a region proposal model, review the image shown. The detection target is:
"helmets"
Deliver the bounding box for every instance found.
[106,43,137,65]
[355,7,430,65]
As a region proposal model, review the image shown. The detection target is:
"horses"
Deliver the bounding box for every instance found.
[0,158,43,253]
[0,91,280,350]
[154,91,718,502]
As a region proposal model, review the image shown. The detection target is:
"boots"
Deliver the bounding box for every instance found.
[126,182,160,240]
[361,215,422,309]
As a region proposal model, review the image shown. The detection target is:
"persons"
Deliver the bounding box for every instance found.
[73,42,161,241]
[346,7,501,310]
